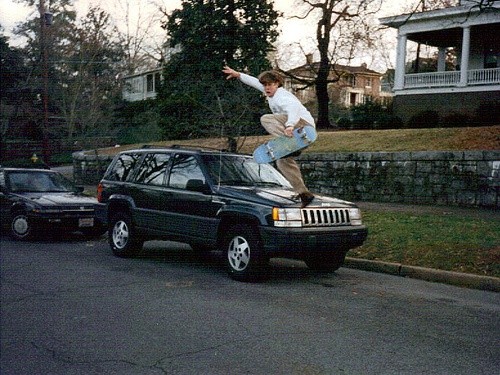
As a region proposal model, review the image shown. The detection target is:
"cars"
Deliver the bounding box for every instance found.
[0,169,109,242]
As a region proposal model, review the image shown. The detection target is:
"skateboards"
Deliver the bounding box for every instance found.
[252,126,317,164]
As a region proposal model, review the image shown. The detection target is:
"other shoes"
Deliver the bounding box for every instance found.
[299,192,315,209]
[279,150,301,158]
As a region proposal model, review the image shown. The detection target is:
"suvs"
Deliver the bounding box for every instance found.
[93,144,370,281]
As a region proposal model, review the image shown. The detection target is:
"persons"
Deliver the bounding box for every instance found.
[221,64,316,209]
